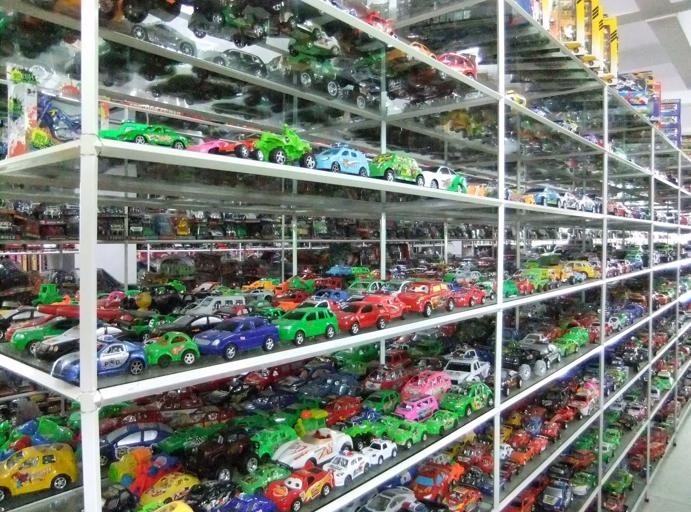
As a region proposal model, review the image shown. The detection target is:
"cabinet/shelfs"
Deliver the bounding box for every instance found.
[2,0,691,512]
